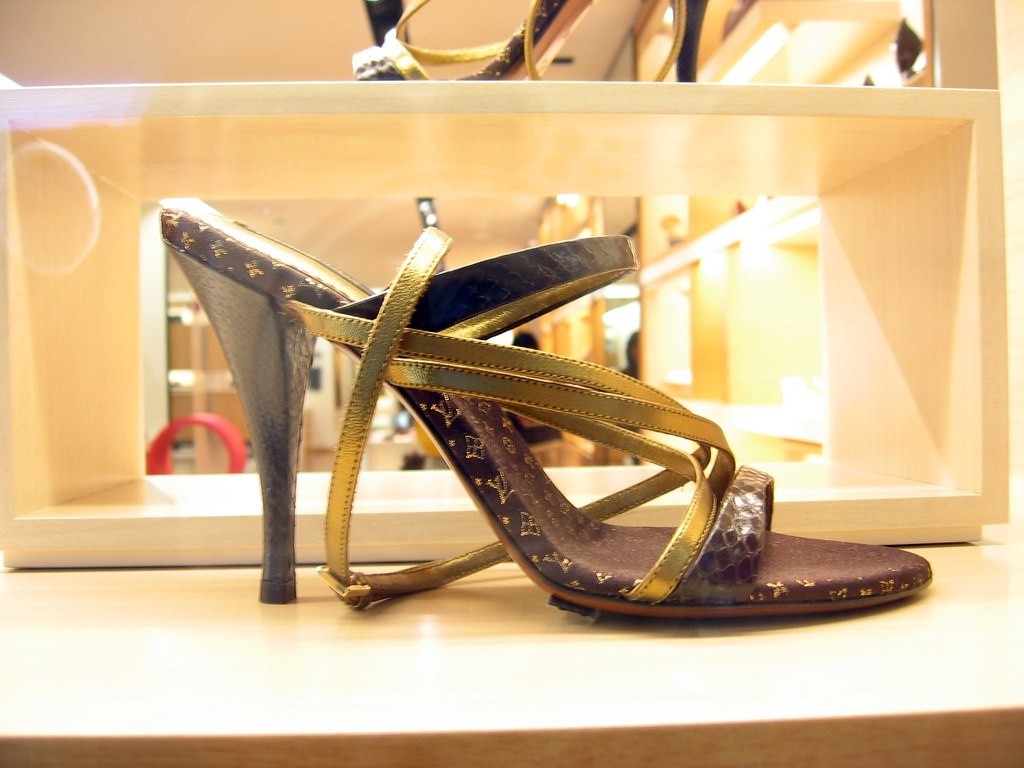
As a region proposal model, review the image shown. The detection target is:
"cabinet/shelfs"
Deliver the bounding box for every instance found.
[2,0,1012,576]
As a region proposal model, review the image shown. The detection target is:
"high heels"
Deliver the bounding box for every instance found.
[160,194,934,615]
[352,0,707,83]
